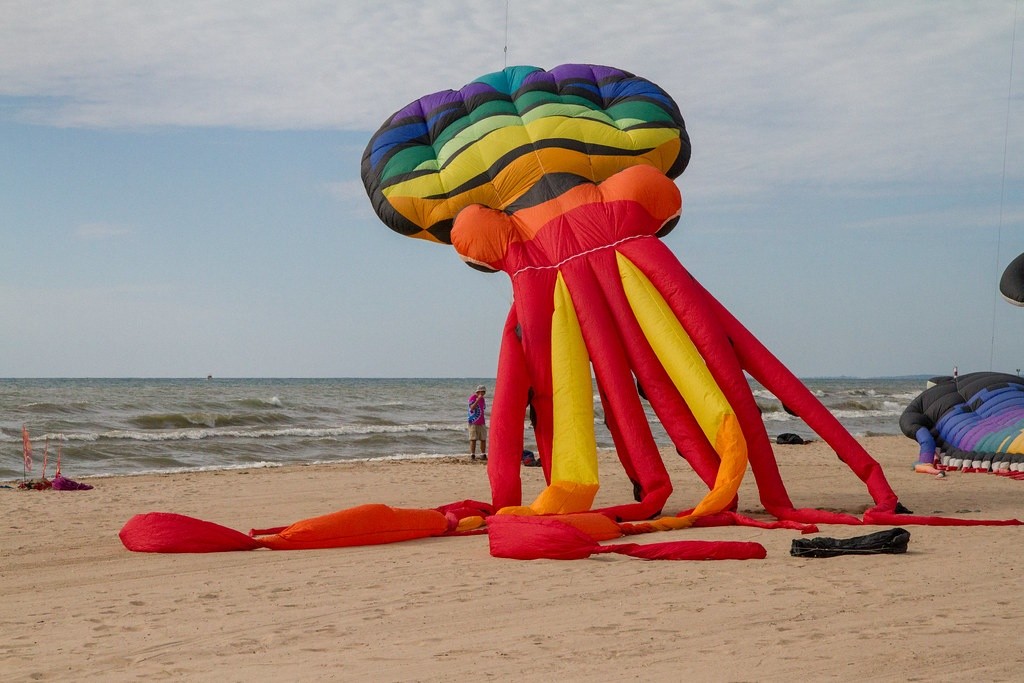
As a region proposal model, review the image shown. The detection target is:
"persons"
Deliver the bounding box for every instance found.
[468,385,488,461]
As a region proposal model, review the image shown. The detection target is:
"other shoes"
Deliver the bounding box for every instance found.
[470,455,475,460]
[480,454,487,460]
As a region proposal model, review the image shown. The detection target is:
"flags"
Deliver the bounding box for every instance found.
[23,426,32,472]
[44,439,47,474]
[57,437,60,477]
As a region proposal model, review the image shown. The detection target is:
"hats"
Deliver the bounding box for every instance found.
[475,385,487,393]
